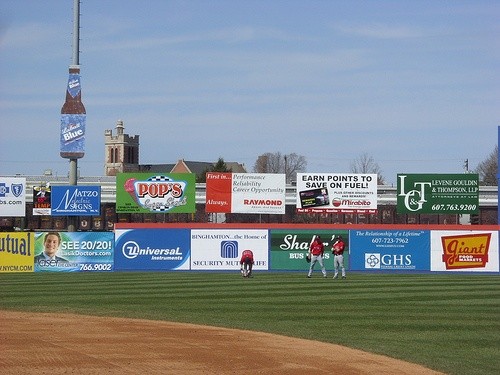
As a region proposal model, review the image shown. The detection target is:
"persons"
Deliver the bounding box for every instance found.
[308,235,327,278]
[331,236,347,279]
[240,250,254,277]
[34,232,68,263]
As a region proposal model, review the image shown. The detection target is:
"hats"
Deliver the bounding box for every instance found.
[336,235,342,239]
[316,236,321,240]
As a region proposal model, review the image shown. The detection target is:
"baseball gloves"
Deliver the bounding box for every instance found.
[306,256,311,263]
